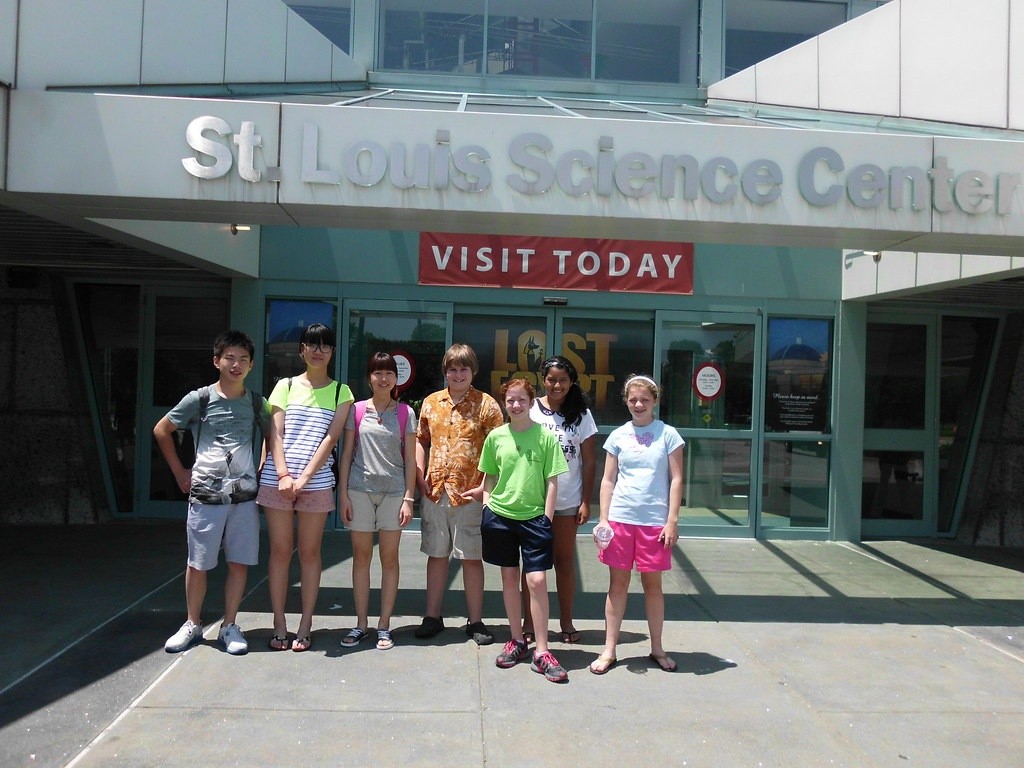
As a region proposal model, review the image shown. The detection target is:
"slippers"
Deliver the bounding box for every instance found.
[590,656,616,674]
[649,654,677,672]
[377,629,394,650]
[268,634,289,650]
[562,631,581,644]
[292,636,312,652]
[340,628,369,647]
[523,632,535,644]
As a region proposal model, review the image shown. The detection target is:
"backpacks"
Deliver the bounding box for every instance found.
[171,385,262,502]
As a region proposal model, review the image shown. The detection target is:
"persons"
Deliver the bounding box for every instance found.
[256,323,354,651]
[339,352,416,651]
[589,376,686,676]
[416,344,504,646]
[154,330,271,654]
[478,355,599,681]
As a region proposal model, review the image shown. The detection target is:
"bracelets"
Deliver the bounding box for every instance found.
[403,498,414,501]
[278,473,290,481]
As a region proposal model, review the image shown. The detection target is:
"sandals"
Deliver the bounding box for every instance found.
[466,617,494,644]
[414,616,444,639]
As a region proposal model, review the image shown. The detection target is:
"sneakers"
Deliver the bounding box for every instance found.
[495,637,530,668]
[530,650,567,682]
[217,623,248,655]
[164,620,204,653]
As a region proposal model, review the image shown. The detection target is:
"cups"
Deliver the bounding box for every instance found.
[596,527,614,550]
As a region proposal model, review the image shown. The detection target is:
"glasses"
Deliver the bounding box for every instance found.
[302,343,335,353]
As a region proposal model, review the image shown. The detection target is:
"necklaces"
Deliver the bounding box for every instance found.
[373,398,392,424]
